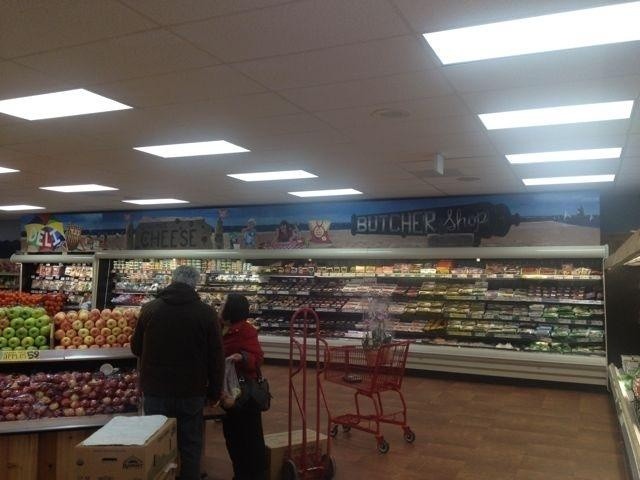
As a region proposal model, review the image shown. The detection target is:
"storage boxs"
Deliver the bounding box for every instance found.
[264,427,331,480]
[154,452,184,480]
[74,410,180,480]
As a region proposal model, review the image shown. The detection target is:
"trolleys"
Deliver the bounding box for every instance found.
[280,306,335,479]
[324,336,415,454]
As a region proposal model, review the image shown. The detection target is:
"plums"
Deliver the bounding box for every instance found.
[0,371,140,422]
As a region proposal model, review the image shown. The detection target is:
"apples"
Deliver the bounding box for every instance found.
[230,387,241,397]
[0,306,51,350]
[53,307,140,348]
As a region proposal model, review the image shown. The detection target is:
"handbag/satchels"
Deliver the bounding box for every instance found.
[222,380,270,413]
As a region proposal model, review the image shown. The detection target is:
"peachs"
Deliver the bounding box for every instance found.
[42,293,64,318]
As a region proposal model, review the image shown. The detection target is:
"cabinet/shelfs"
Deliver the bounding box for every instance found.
[101,266,261,342]
[0,269,25,296]
[253,269,608,389]
[1,341,151,480]
[23,263,100,311]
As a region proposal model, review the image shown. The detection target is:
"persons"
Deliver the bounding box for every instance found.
[129,265,225,480]
[219,293,271,479]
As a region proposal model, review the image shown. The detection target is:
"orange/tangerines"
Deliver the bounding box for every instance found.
[0,291,42,307]
